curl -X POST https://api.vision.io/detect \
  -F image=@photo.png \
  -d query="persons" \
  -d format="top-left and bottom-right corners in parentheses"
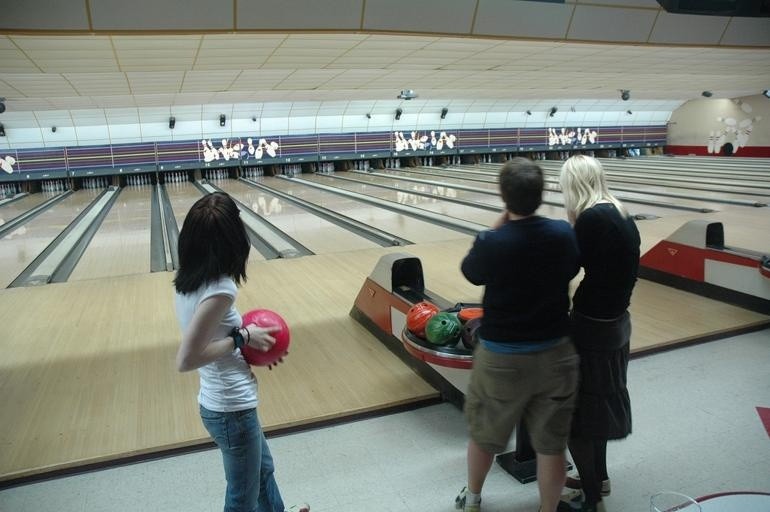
top-left (454, 156), bottom-right (581, 512)
top-left (552, 155), bottom-right (642, 512)
top-left (175, 191), bottom-right (310, 512)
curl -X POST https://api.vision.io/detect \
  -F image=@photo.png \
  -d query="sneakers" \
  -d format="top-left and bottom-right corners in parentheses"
top-left (538, 488), bottom-right (587, 512)
top-left (560, 486), bottom-right (608, 512)
top-left (284, 503), bottom-right (310, 512)
top-left (455, 486), bottom-right (482, 511)
top-left (563, 474), bottom-right (612, 496)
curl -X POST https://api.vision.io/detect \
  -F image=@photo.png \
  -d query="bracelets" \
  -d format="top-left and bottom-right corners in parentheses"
top-left (244, 327), bottom-right (251, 345)
top-left (226, 327), bottom-right (245, 349)
